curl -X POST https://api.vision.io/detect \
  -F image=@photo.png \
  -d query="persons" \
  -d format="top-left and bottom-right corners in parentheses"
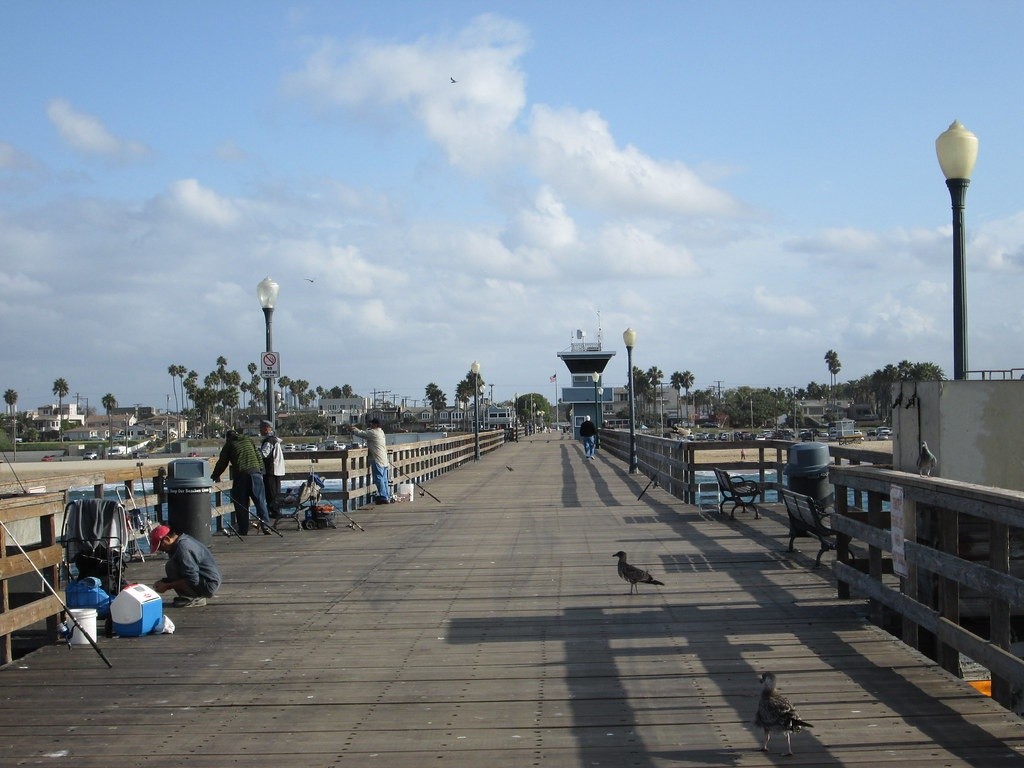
top-left (149, 525), bottom-right (221, 608)
top-left (258, 420), bottom-right (285, 518)
top-left (580, 415), bottom-right (596, 460)
top-left (350, 417), bottom-right (390, 503)
top-left (210, 430), bottom-right (272, 535)
top-left (740, 449), bottom-right (745, 460)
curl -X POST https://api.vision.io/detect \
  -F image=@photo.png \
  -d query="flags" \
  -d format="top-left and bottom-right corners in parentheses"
top-left (550, 374), bottom-right (556, 383)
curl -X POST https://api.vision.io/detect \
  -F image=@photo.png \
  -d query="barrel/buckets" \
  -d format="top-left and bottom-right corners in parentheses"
top-left (64, 608), bottom-right (98, 645)
top-left (400, 484), bottom-right (414, 501)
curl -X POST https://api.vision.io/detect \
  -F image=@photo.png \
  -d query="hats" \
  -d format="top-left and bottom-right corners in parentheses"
top-left (149, 525), bottom-right (170, 554)
top-left (257, 419), bottom-right (273, 428)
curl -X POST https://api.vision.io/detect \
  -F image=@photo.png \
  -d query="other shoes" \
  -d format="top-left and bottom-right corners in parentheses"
top-left (377, 499), bottom-right (390, 504)
top-left (234, 531), bottom-right (246, 536)
top-left (261, 527), bottom-right (272, 535)
top-left (184, 597), bottom-right (207, 607)
top-left (173, 596), bottom-right (190, 605)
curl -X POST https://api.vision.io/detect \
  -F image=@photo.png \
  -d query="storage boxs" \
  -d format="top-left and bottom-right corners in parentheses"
top-left (110, 583), bottom-right (163, 638)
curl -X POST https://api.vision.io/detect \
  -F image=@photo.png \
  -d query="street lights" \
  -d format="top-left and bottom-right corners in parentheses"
top-left (511, 397), bottom-right (515, 411)
top-left (934, 119), bottom-right (979, 381)
top-left (622, 327), bottom-right (638, 474)
top-left (598, 387), bottom-right (604, 428)
top-left (533, 403), bottom-right (536, 434)
top-left (591, 371), bottom-right (599, 448)
top-left (256, 275), bottom-right (280, 436)
top-left (479, 385), bottom-right (486, 431)
top-left (515, 393), bottom-right (520, 443)
top-left (471, 361), bottom-right (480, 460)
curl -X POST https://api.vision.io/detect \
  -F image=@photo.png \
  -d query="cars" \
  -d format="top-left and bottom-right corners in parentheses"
top-left (688, 426), bottom-right (836, 441)
top-left (854, 426), bottom-right (893, 441)
top-left (107, 448), bottom-right (123, 456)
top-left (88, 436), bottom-right (105, 441)
top-left (41, 455), bottom-right (52, 461)
top-left (82, 452), bottom-right (96, 460)
top-left (282, 440), bottom-right (368, 452)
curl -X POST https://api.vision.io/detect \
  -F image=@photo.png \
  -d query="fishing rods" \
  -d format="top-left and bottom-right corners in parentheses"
top-left (215, 485), bottom-right (284, 538)
top-left (1, 519), bottom-right (113, 670)
top-left (636, 391), bottom-right (717, 502)
top-left (284, 460), bottom-right (365, 532)
top-left (0, 445), bottom-right (77, 582)
top-left (356, 436), bottom-right (442, 503)
top-left (210, 504), bottom-right (244, 542)
top-left (136, 443), bottom-right (152, 532)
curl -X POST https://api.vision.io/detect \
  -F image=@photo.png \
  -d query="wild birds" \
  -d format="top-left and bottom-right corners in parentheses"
top-left (304, 277), bottom-right (317, 283)
top-left (530, 440), bottom-right (532, 443)
top-left (752, 672), bottom-right (815, 757)
top-left (505, 465), bottom-right (514, 472)
top-left (612, 551), bottom-right (665, 595)
top-left (450, 76), bottom-right (459, 83)
top-left (546, 440), bottom-right (550, 443)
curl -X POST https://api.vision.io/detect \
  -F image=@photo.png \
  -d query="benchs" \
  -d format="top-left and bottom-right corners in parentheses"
top-left (781, 488), bottom-right (855, 567)
top-left (836, 435), bottom-right (864, 445)
top-left (713, 467), bottom-right (761, 520)
